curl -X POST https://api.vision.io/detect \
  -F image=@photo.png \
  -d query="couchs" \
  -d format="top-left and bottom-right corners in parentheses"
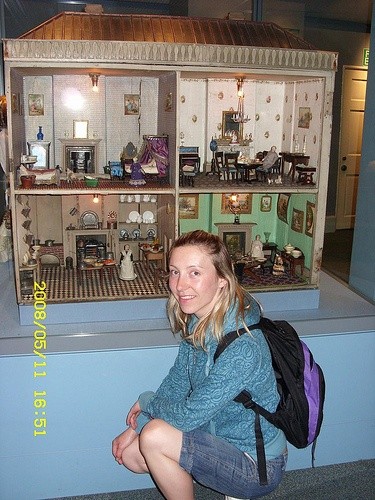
top-left (16, 164), bottom-right (61, 187)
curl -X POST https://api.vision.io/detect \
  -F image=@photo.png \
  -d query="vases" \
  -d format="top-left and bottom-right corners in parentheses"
top-left (37, 126), bottom-right (44, 140)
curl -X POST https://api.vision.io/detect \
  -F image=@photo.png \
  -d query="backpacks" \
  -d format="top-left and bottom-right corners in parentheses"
top-left (214, 316), bottom-right (327, 448)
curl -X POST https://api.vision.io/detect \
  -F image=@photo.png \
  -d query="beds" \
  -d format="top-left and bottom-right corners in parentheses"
top-left (123, 134), bottom-right (169, 183)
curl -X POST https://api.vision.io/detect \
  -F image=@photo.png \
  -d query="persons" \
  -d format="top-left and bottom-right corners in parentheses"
top-left (118, 244), bottom-right (137, 280)
top-left (112, 230), bottom-right (288, 500)
top-left (251, 234), bottom-right (265, 262)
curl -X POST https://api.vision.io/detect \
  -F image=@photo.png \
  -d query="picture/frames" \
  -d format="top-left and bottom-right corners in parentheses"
top-left (222, 110), bottom-right (243, 144)
top-left (276, 193), bottom-right (292, 225)
top-left (72, 119), bottom-right (89, 139)
top-left (28, 93), bottom-right (44, 116)
top-left (260, 195), bottom-right (272, 212)
top-left (297, 106), bottom-right (310, 129)
top-left (178, 193), bottom-right (199, 220)
top-left (290, 206), bottom-right (305, 233)
top-left (305, 200), bottom-right (316, 237)
top-left (221, 193), bottom-right (253, 214)
top-left (124, 94), bottom-right (139, 116)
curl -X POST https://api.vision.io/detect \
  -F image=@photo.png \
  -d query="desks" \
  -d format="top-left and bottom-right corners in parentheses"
top-left (27, 140), bottom-right (51, 170)
top-left (262, 242), bottom-right (278, 260)
top-left (19, 256), bottom-right (42, 297)
top-left (272, 246), bottom-right (305, 278)
top-left (231, 158), bottom-right (265, 184)
top-left (229, 255), bottom-right (267, 275)
top-left (212, 145), bottom-right (253, 175)
top-left (179, 145), bottom-right (200, 175)
top-left (279, 152), bottom-right (310, 183)
top-left (139, 247), bottom-right (164, 276)
top-left (78, 257), bottom-right (115, 286)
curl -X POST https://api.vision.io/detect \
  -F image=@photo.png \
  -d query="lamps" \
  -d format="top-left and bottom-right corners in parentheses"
top-left (231, 76), bottom-right (252, 123)
top-left (89, 72), bottom-right (100, 92)
top-left (21, 207), bottom-right (31, 219)
top-left (69, 206), bottom-right (79, 216)
top-left (93, 194), bottom-right (99, 203)
top-left (227, 193), bottom-right (242, 215)
top-left (25, 234), bottom-right (35, 245)
top-left (22, 220), bottom-right (32, 232)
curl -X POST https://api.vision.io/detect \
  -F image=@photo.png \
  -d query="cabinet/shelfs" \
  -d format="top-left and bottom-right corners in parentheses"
top-left (118, 201), bottom-right (159, 242)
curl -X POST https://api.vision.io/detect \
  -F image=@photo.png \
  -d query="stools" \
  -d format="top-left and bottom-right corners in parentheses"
top-left (295, 164), bottom-right (317, 186)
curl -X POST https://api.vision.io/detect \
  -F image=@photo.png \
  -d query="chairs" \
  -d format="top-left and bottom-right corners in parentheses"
top-left (153, 268), bottom-right (170, 292)
top-left (233, 262), bottom-right (246, 282)
top-left (256, 157), bottom-right (282, 182)
top-left (215, 151), bottom-right (234, 183)
top-left (108, 160), bottom-right (123, 181)
top-left (179, 155), bottom-right (202, 187)
top-left (223, 152), bottom-right (247, 186)
